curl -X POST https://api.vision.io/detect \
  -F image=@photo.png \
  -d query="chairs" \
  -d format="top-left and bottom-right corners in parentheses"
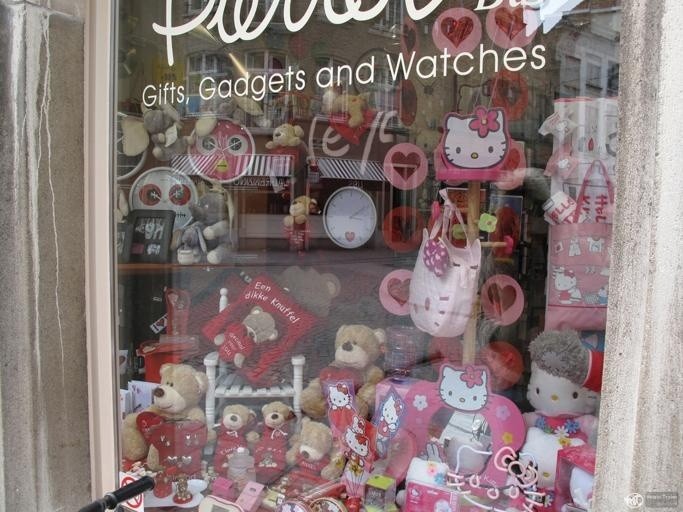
top-left (203, 286), bottom-right (306, 455)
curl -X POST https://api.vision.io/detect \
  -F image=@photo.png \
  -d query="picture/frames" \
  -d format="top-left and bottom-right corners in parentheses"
top-left (121, 209), bottom-right (175, 264)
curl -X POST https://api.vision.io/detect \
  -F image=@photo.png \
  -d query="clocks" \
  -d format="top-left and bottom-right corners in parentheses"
top-left (128, 166), bottom-right (199, 232)
top-left (322, 186), bottom-right (378, 251)
top-left (117, 112), bottom-right (147, 180)
top-left (187, 117), bottom-right (256, 184)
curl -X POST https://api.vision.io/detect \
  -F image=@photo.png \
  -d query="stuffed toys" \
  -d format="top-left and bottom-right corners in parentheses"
top-left (518, 329), bottom-right (603, 511)
top-left (121, 362), bottom-right (206, 481)
top-left (196, 88), bottom-right (264, 138)
top-left (139, 94), bottom-right (194, 163)
top-left (321, 88), bottom-right (370, 128)
top-left (117, 304), bottom-right (605, 511)
top-left (180, 190), bottom-right (233, 266)
top-left (208, 400), bottom-right (343, 512)
top-left (265, 124), bottom-right (309, 178)
top-left (299, 324), bottom-right (386, 420)
top-left (214, 305), bottom-right (279, 368)
top-left (283, 194), bottom-right (322, 252)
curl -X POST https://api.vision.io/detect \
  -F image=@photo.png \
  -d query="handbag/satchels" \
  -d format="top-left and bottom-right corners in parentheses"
top-left (543, 223), bottom-right (615, 333)
top-left (408, 231), bottom-right (482, 338)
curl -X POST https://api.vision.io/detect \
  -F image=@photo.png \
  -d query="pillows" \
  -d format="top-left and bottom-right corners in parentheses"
top-left (202, 275), bottom-right (317, 389)
